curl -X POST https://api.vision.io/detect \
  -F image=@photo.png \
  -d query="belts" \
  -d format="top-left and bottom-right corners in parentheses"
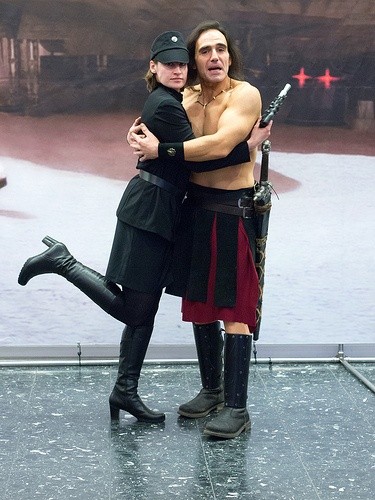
top-left (188, 196), bottom-right (256, 219)
top-left (138, 169), bottom-right (188, 204)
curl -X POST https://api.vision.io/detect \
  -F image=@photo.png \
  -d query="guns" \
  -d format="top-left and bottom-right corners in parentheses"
top-left (260, 83), bottom-right (291, 130)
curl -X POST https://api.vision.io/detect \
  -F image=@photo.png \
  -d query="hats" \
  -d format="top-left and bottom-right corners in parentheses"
top-left (150, 31), bottom-right (191, 63)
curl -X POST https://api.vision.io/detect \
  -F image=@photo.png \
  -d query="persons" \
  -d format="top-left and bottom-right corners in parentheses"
top-left (127, 20), bottom-right (262, 438)
top-left (18, 32), bottom-right (273, 425)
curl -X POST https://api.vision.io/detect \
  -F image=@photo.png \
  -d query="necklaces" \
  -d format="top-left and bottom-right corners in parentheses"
top-left (194, 79), bottom-right (235, 110)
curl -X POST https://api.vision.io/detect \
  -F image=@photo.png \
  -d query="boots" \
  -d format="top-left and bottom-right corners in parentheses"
top-left (177, 323), bottom-right (225, 418)
top-left (109, 324), bottom-right (165, 422)
top-left (203, 334), bottom-right (251, 436)
top-left (17, 236), bottom-right (121, 322)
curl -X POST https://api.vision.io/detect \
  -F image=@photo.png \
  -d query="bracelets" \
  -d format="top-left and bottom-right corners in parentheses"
top-left (158, 141), bottom-right (185, 162)
